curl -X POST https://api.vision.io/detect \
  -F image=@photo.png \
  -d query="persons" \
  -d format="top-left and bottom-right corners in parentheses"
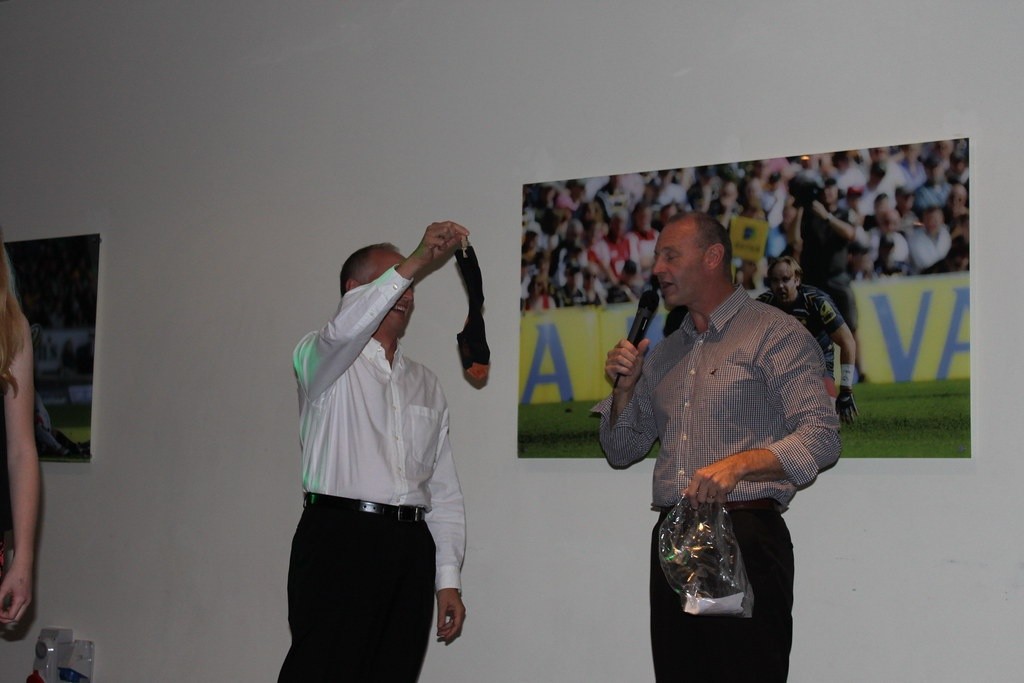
top-left (759, 251), bottom-right (859, 425)
top-left (589, 210), bottom-right (843, 681)
top-left (783, 171), bottom-right (871, 383)
top-left (521, 137), bottom-right (970, 310)
top-left (260, 219), bottom-right (473, 682)
top-left (1, 235), bottom-right (39, 633)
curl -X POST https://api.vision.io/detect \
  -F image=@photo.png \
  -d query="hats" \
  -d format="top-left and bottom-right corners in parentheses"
top-left (822, 172), bottom-right (837, 184)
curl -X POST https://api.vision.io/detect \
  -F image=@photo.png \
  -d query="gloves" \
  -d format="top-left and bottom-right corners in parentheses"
top-left (835, 385), bottom-right (858, 426)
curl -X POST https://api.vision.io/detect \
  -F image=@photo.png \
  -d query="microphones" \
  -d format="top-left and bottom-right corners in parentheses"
top-left (618, 290), bottom-right (659, 377)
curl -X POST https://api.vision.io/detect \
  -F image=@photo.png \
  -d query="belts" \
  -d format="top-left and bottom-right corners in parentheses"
top-left (304, 492), bottom-right (425, 521)
top-left (671, 497), bottom-right (772, 512)
